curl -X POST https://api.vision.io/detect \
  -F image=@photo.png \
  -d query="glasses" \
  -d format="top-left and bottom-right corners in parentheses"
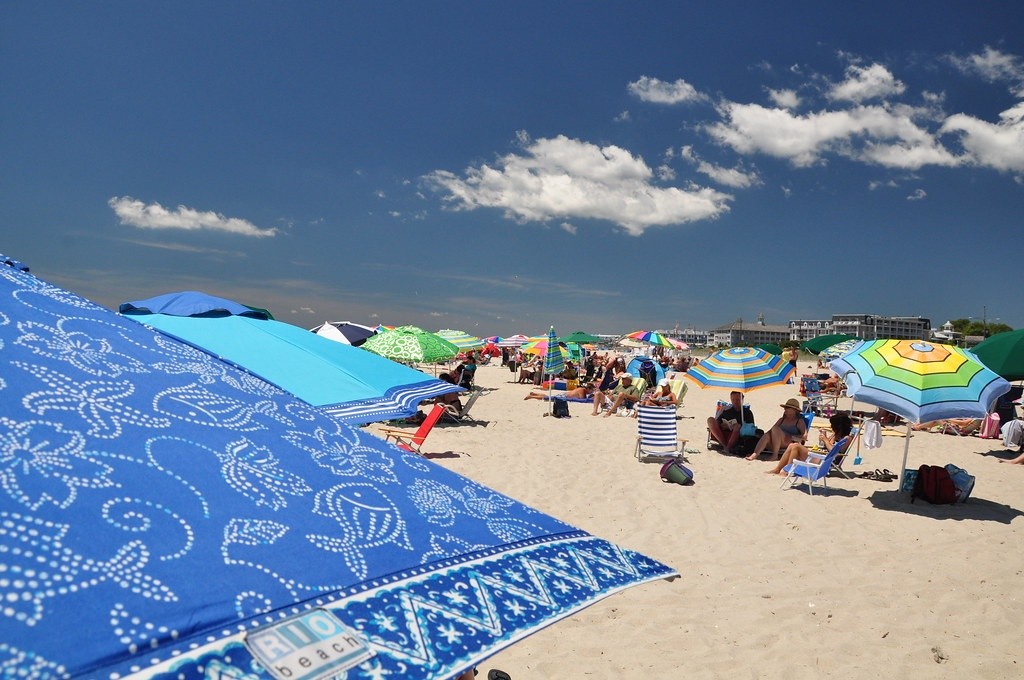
top-left (783, 406), bottom-right (790, 409)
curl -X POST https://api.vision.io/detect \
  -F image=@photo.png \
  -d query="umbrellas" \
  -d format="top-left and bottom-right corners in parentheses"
top-left (801, 333), bottom-right (1012, 495)
top-left (969, 329), bottom-right (1024, 383)
top-left (683, 346), bottom-right (797, 424)
top-left (1, 253), bottom-right (682, 679)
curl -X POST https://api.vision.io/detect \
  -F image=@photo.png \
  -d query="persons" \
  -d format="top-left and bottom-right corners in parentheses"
top-left (782, 347), bottom-right (791, 364)
top-left (439, 364), bottom-right (465, 385)
top-left (764, 413), bottom-right (853, 476)
top-left (872, 408), bottom-right (892, 422)
top-left (504, 348), bottom-right (754, 455)
top-left (788, 347), bottom-right (798, 377)
top-left (461, 356), bottom-right (477, 385)
top-left (906, 419), bottom-right (971, 436)
top-left (745, 398), bottom-right (807, 461)
top-left (819, 373), bottom-right (839, 392)
top-left (434, 373), bottom-right (462, 418)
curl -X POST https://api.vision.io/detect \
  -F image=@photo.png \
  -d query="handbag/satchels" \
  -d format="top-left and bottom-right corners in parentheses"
top-left (944, 462), bottom-right (975, 505)
top-left (902, 463), bottom-right (919, 493)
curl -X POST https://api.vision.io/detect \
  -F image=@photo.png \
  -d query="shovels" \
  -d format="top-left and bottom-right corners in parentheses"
top-left (854, 414), bottom-right (862, 465)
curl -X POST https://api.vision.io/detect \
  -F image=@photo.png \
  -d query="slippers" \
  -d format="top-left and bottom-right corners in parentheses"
top-left (684, 448), bottom-right (700, 453)
top-left (859, 468), bottom-right (898, 482)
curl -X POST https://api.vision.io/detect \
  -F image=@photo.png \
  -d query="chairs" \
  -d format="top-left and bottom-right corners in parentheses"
top-left (651, 379), bottom-right (689, 418)
top-left (457, 368), bottom-right (476, 391)
top-left (632, 402), bottom-right (691, 464)
top-left (705, 399), bottom-right (752, 449)
top-left (801, 377), bottom-right (841, 417)
top-left (440, 386), bottom-right (491, 428)
top-left (826, 422), bottom-right (867, 480)
top-left (778, 432), bottom-right (849, 494)
top-left (762, 410), bottom-right (819, 454)
top-left (377, 401), bottom-right (460, 460)
top-left (601, 377), bottom-right (649, 413)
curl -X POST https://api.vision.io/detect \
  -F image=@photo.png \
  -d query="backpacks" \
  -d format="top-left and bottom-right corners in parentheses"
top-left (553, 396), bottom-right (571, 418)
top-left (910, 464), bottom-right (957, 506)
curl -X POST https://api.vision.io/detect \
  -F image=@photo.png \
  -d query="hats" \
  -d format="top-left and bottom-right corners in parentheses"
top-left (780, 398), bottom-right (803, 412)
top-left (658, 378), bottom-right (670, 387)
top-left (621, 372), bottom-right (633, 381)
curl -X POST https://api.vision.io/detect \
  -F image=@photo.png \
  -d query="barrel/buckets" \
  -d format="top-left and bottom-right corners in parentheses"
top-left (659, 459), bottom-right (689, 483)
